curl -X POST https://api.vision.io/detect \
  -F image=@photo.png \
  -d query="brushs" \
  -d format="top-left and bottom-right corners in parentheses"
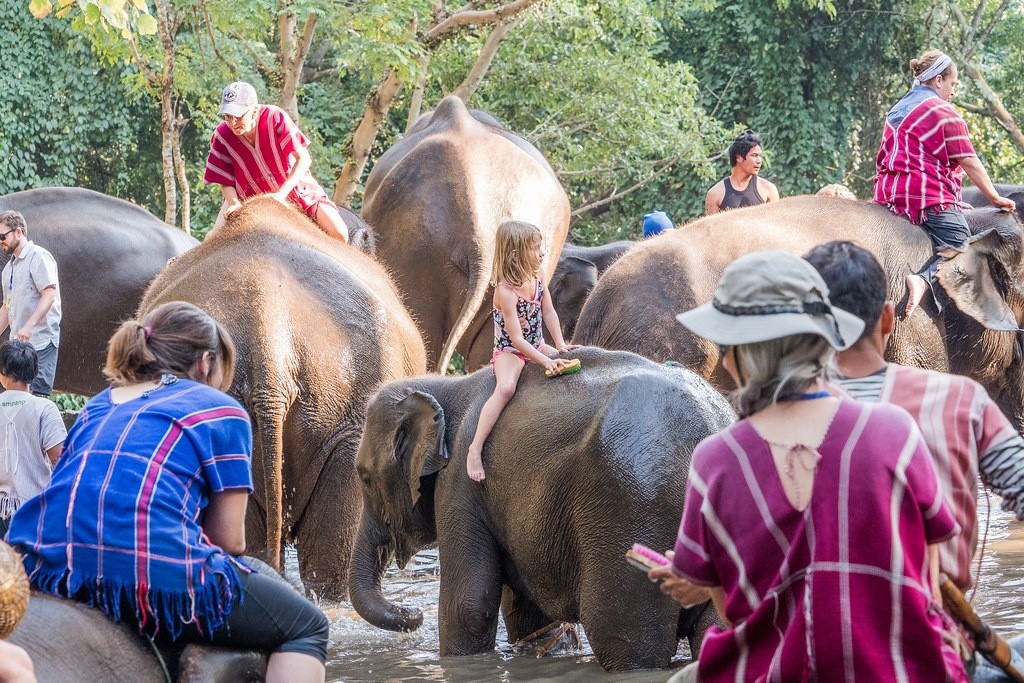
top-left (625, 543), bottom-right (671, 580)
top-left (1001, 206), bottom-right (1012, 212)
top-left (545, 358), bottom-right (581, 377)
top-left (225, 208), bottom-right (236, 220)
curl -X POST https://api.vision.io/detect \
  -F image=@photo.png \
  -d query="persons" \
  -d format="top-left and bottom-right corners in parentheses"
top-left (0, 638), bottom-right (37, 683)
top-left (466, 219), bottom-right (567, 482)
top-left (800, 242), bottom-right (1024, 673)
top-left (0, 339), bottom-right (68, 539)
top-left (0, 210), bottom-right (62, 398)
top-left (203, 81), bottom-right (349, 244)
top-left (705, 130), bottom-right (779, 217)
top-left (643, 210), bottom-right (674, 238)
top-left (648, 250), bottom-right (971, 683)
top-left (874, 50), bottom-right (1017, 318)
top-left (5, 302), bottom-right (329, 683)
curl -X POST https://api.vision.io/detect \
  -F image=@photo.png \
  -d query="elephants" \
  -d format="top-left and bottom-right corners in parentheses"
top-left (348, 347), bottom-right (743, 666)
top-left (0, 95), bottom-right (1024, 683)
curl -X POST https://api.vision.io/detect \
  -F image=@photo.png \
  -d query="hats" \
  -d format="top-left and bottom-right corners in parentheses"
top-left (217, 81), bottom-right (258, 117)
top-left (643, 210), bottom-right (674, 238)
top-left (676, 250), bottom-right (865, 351)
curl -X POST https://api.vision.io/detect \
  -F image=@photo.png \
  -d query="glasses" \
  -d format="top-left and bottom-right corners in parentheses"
top-left (719, 346), bottom-right (727, 356)
top-left (0, 229), bottom-right (16, 241)
top-left (221, 110), bottom-right (249, 121)
